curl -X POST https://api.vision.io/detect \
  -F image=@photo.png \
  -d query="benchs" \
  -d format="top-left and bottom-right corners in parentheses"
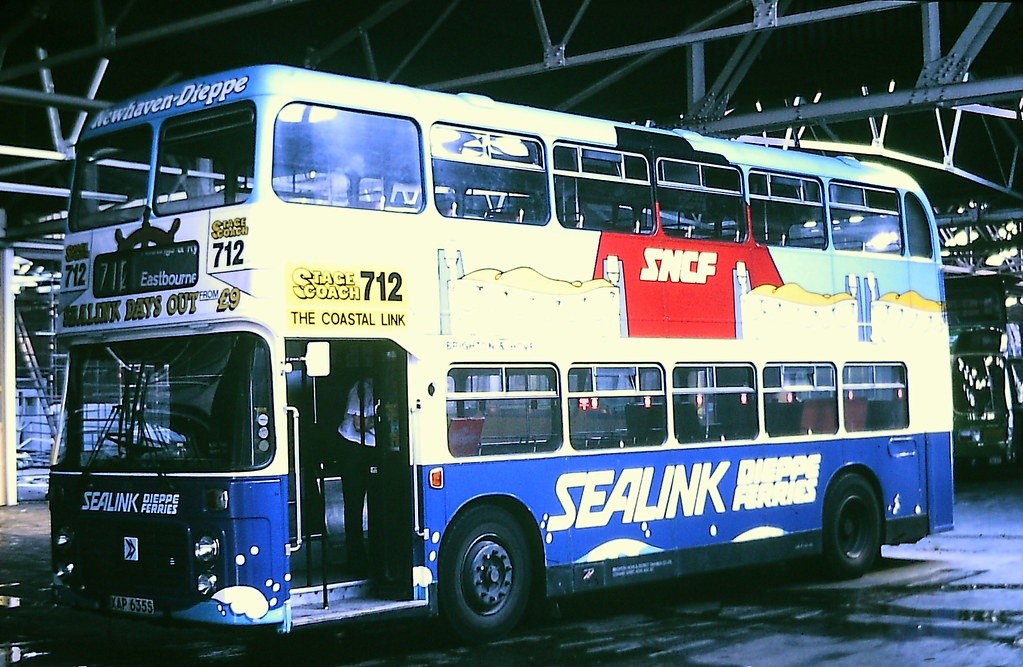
top-left (343, 191), bottom-right (901, 255)
top-left (446, 396), bottom-right (909, 457)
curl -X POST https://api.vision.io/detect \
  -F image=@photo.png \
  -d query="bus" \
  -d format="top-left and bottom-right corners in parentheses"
top-left (45, 64), bottom-right (957, 646)
top-left (940, 267), bottom-right (1023, 465)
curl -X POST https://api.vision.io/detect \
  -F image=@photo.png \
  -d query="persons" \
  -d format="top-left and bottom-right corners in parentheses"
top-left (338, 377), bottom-right (377, 559)
